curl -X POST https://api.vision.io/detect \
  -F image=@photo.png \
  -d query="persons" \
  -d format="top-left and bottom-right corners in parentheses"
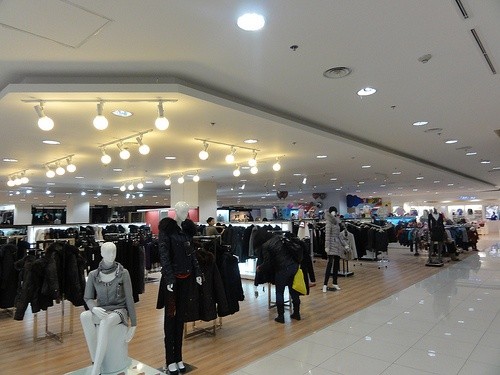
top-left (156, 201), bottom-right (202, 372)
top-left (80, 242), bottom-right (136, 375)
top-left (325, 206), bottom-right (351, 292)
top-left (206, 217), bottom-right (226, 235)
top-left (491, 212), bottom-right (499, 221)
top-left (428, 208), bottom-right (445, 263)
top-left (254, 230), bottom-right (303, 323)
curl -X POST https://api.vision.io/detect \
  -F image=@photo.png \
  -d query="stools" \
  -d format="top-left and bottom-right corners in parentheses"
top-left (94, 322), bottom-right (132, 373)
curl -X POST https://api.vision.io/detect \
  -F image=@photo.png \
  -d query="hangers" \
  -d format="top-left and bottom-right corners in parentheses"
top-left (366, 223), bottom-right (384, 233)
top-left (192, 238), bottom-right (210, 255)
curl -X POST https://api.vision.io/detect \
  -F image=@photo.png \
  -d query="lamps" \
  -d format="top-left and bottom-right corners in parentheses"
top-left (120, 179), bottom-right (144, 192)
top-left (233, 166), bottom-right (241, 178)
top-left (7, 168), bottom-right (29, 186)
top-left (198, 143), bottom-right (209, 160)
top-left (34, 101), bottom-right (55, 131)
top-left (164, 169), bottom-right (200, 185)
top-left (250, 165), bottom-right (258, 175)
top-left (273, 161), bottom-right (280, 171)
top-left (154, 100), bottom-right (170, 131)
top-left (117, 140), bottom-right (131, 160)
top-left (43, 154), bottom-right (76, 178)
top-left (99, 147), bottom-right (112, 165)
top-left (135, 134), bottom-right (152, 155)
top-left (247, 150), bottom-right (259, 167)
top-left (226, 147), bottom-right (237, 164)
top-left (92, 102), bottom-right (109, 131)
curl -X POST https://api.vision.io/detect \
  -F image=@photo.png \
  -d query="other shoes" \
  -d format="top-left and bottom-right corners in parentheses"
top-left (275, 314), bottom-right (284, 323)
top-left (291, 311), bottom-right (300, 319)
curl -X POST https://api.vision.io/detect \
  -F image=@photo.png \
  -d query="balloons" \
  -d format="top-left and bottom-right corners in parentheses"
top-left (277, 191), bottom-right (289, 201)
top-left (310, 193), bottom-right (327, 208)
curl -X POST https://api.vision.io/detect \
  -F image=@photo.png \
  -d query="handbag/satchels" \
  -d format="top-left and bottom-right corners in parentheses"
top-left (279, 235), bottom-right (304, 265)
top-left (292, 264), bottom-right (307, 295)
